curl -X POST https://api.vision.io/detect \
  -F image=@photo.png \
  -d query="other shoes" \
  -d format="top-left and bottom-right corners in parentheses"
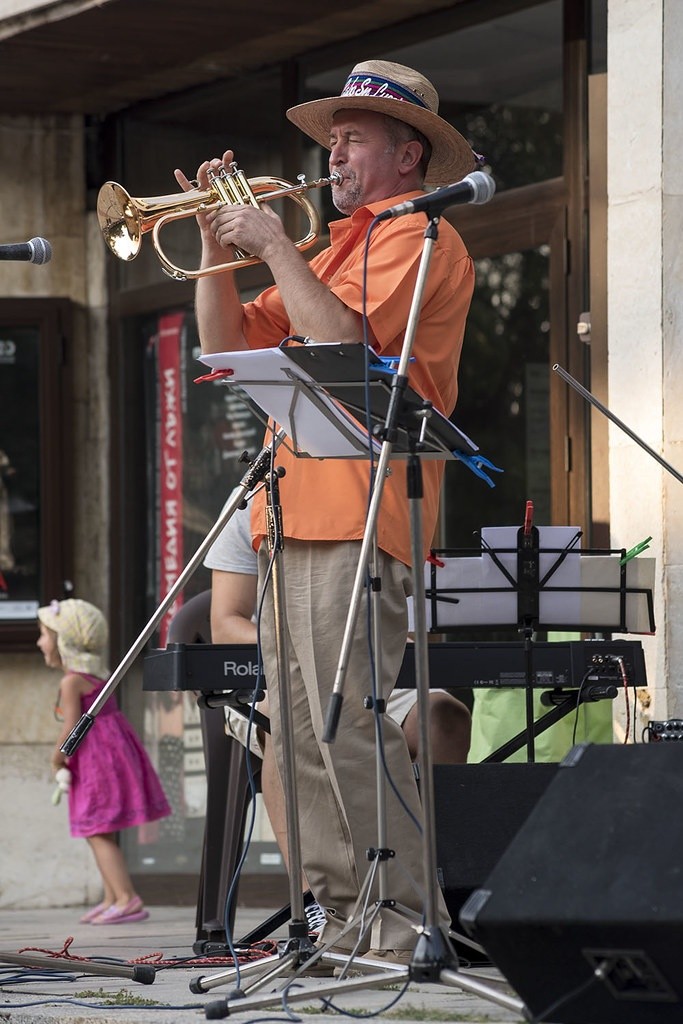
top-left (332, 949), bottom-right (414, 979)
top-left (277, 942), bottom-right (366, 978)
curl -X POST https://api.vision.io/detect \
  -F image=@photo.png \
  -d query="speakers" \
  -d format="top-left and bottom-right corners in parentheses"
top-left (411, 737), bottom-right (683, 1024)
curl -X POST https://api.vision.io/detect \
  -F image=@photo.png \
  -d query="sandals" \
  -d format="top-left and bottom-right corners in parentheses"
top-left (91, 896), bottom-right (149, 925)
top-left (79, 903), bottom-right (110, 924)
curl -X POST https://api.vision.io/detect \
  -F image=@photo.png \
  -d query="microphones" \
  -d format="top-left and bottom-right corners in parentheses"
top-left (0, 237), bottom-right (52, 265)
top-left (376, 170), bottom-right (496, 221)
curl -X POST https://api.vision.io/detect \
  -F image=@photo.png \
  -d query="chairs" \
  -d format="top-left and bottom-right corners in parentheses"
top-left (169, 589), bottom-right (273, 952)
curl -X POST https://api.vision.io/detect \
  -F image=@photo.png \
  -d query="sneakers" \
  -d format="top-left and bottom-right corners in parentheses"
top-left (297, 890), bottom-right (327, 939)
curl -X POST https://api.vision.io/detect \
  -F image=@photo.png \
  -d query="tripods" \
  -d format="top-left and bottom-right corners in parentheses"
top-left (188, 342), bottom-right (532, 1021)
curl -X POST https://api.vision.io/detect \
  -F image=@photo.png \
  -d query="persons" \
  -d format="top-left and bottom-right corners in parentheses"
top-left (201, 478), bottom-right (470, 938)
top-left (35, 599), bottom-right (173, 925)
top-left (172, 60), bottom-right (475, 982)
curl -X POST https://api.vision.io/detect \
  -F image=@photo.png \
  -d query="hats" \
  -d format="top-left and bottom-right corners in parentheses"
top-left (286, 60), bottom-right (475, 187)
top-left (37, 598), bottom-right (112, 681)
